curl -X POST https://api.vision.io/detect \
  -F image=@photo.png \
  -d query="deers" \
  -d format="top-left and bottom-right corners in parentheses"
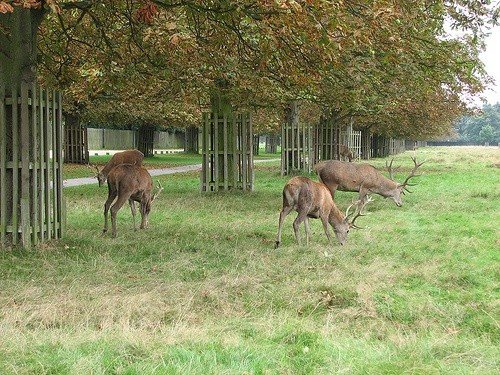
top-left (85, 149), bottom-right (144, 187)
top-left (276, 175), bottom-right (376, 247)
top-left (314, 153), bottom-right (425, 215)
top-left (102, 163), bottom-right (165, 239)
